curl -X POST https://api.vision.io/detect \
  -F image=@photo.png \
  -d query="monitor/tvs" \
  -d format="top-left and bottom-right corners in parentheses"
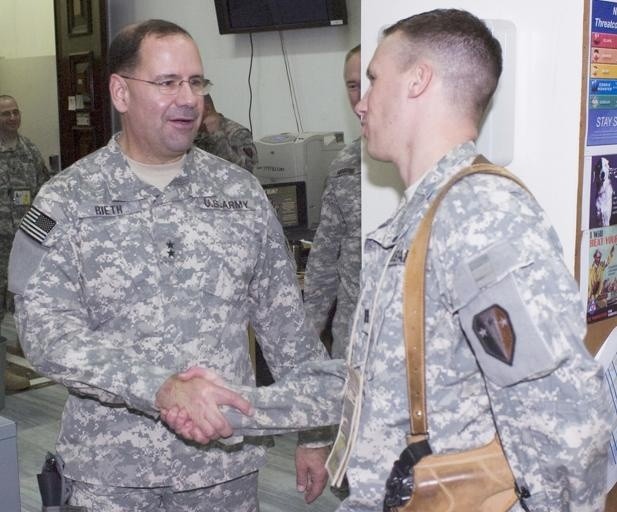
top-left (214, 0), bottom-right (348, 35)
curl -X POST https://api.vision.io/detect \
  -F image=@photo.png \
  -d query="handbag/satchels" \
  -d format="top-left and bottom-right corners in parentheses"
top-left (383, 440), bottom-right (522, 512)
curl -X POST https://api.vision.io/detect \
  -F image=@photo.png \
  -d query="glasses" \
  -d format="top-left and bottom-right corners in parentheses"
top-left (123, 76), bottom-right (214, 96)
top-left (0, 110), bottom-right (21, 117)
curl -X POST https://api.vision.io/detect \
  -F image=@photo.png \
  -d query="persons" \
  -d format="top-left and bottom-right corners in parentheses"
top-left (159, 10), bottom-right (612, 511)
top-left (1, 94), bottom-right (52, 391)
top-left (7, 20), bottom-right (336, 511)
